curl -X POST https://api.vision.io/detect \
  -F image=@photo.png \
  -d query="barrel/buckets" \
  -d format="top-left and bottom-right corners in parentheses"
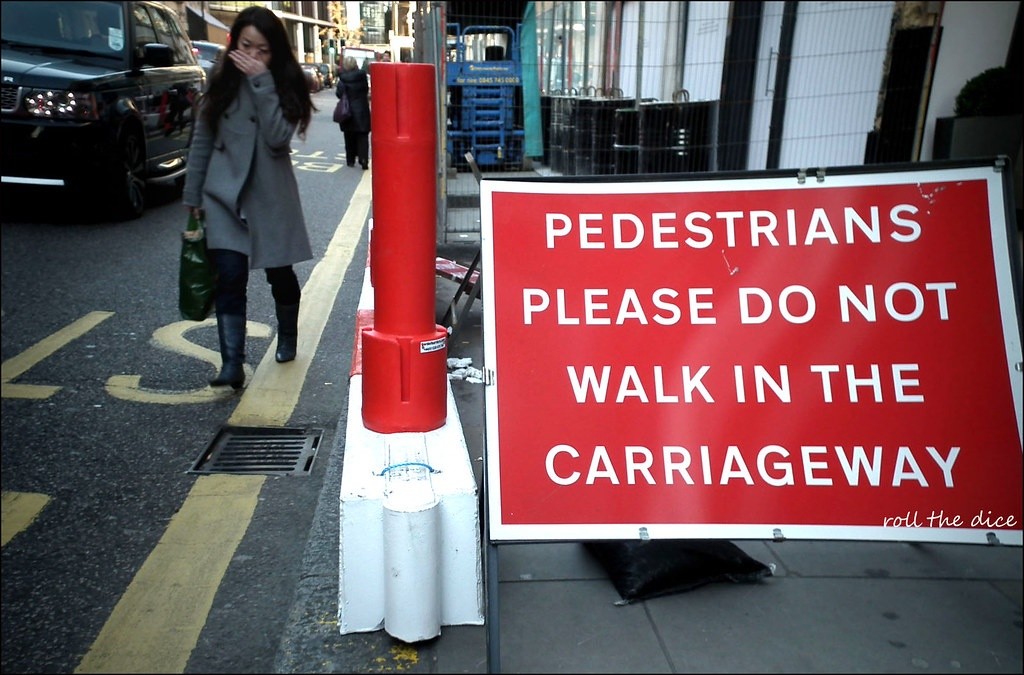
top-left (485, 46), bottom-right (504, 61)
top-left (541, 96), bottom-right (549, 166)
top-left (638, 89), bottom-right (716, 174)
top-left (560, 98), bottom-right (574, 175)
top-left (550, 97), bottom-right (560, 171)
top-left (591, 98), bottom-right (636, 174)
top-left (613, 108), bottom-right (640, 174)
top-left (573, 98), bottom-right (593, 174)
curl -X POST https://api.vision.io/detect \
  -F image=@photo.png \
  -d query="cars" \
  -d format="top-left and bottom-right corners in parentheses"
top-left (189, 41), bottom-right (226, 76)
top-left (299, 63), bottom-right (327, 92)
top-left (0, 0), bottom-right (207, 220)
top-left (314, 63), bottom-right (333, 88)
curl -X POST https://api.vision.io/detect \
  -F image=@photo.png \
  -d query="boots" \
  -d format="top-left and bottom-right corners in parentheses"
top-left (275, 301), bottom-right (297, 363)
top-left (209, 315), bottom-right (245, 389)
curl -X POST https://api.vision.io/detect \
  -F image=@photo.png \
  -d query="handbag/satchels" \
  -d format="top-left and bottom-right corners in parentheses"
top-left (334, 95), bottom-right (351, 123)
top-left (178, 210), bottom-right (216, 322)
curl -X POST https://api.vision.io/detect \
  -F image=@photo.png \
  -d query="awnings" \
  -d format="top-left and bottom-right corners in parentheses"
top-left (186, 1), bottom-right (230, 32)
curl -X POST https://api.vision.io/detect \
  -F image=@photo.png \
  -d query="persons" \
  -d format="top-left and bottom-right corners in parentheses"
top-left (383, 51), bottom-right (390, 62)
top-left (335, 55), bottom-right (370, 170)
top-left (182, 6), bottom-right (313, 391)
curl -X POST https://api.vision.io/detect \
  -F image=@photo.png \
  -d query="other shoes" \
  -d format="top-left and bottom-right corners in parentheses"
top-left (362, 159), bottom-right (368, 170)
